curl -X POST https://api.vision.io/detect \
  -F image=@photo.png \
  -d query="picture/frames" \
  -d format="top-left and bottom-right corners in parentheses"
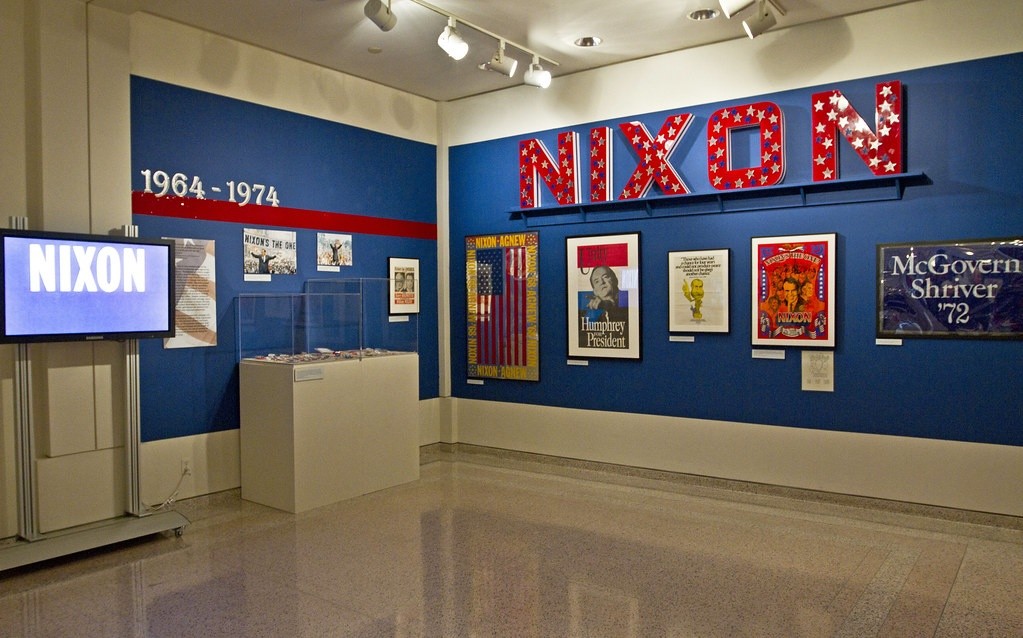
top-left (751, 232), bottom-right (838, 351)
top-left (565, 231), bottom-right (643, 360)
top-left (387, 256), bottom-right (420, 316)
top-left (667, 247), bottom-right (730, 335)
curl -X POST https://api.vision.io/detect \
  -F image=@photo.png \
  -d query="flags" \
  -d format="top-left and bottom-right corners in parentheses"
top-left (477, 247), bottom-right (527, 366)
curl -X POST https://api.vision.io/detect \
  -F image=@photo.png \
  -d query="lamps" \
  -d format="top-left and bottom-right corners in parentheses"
top-left (719, 0), bottom-right (755, 19)
top-left (364, 0), bottom-right (397, 31)
top-left (524, 55), bottom-right (551, 88)
top-left (438, 16), bottom-right (468, 60)
top-left (742, 0), bottom-right (778, 39)
top-left (490, 39), bottom-right (518, 77)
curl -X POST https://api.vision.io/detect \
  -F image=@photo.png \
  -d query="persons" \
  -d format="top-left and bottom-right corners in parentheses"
top-left (585, 266), bottom-right (628, 322)
top-left (395, 272), bottom-right (414, 292)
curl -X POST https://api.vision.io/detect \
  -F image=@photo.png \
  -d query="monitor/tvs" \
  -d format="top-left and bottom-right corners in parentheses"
top-left (0, 228), bottom-right (176, 344)
